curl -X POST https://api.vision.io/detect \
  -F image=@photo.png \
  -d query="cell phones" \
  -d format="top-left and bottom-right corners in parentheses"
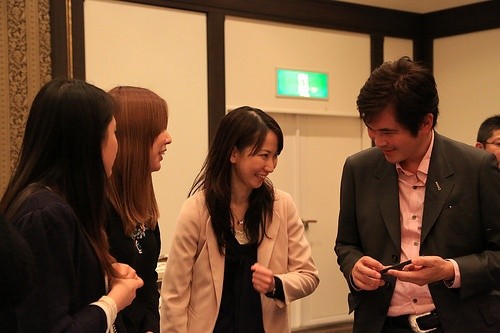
top-left (380, 260), bottom-right (412, 275)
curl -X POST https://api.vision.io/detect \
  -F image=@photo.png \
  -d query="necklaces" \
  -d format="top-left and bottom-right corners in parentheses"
top-left (229, 206), bottom-right (250, 225)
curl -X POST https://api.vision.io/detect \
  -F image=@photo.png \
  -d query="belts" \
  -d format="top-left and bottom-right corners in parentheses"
top-left (382, 308), bottom-right (441, 333)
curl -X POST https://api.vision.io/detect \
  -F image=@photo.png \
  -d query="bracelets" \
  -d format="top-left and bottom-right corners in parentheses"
top-left (265, 275), bottom-right (276, 295)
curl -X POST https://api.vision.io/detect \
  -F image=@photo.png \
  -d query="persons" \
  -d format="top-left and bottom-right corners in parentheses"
top-left (0, 78), bottom-right (144, 333)
top-left (334, 56), bottom-right (500, 333)
top-left (160, 105), bottom-right (320, 332)
top-left (475, 114), bottom-right (500, 166)
top-left (93, 85), bottom-right (172, 333)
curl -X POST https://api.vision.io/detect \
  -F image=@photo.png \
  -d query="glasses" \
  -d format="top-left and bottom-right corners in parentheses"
top-left (480, 141), bottom-right (500, 147)
